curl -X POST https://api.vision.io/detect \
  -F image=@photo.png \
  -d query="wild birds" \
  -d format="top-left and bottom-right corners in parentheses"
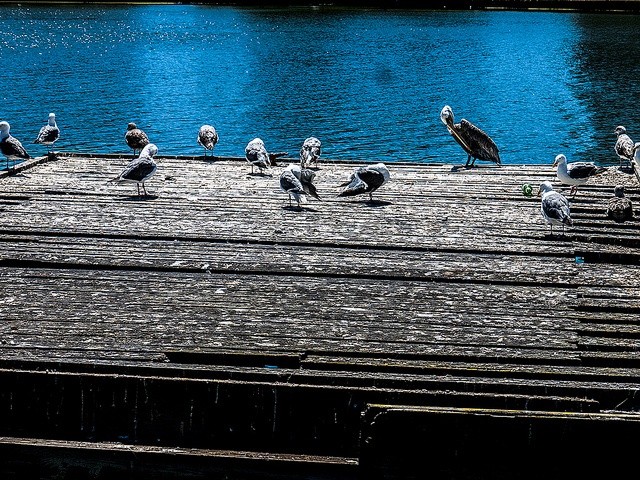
top-left (633, 142), bottom-right (640, 166)
top-left (279, 164), bottom-right (323, 210)
top-left (553, 154), bottom-right (608, 198)
top-left (196, 124), bottom-right (219, 159)
top-left (106, 143), bottom-right (157, 198)
top-left (245, 138), bottom-right (271, 174)
top-left (537, 181), bottom-right (574, 236)
top-left (441, 104), bottom-right (501, 169)
top-left (605, 185), bottom-right (633, 221)
top-left (613, 124), bottom-right (635, 169)
top-left (0, 120), bottom-right (35, 171)
top-left (301, 137), bottom-right (322, 168)
top-left (336, 162), bottom-right (389, 203)
top-left (125, 122), bottom-right (149, 156)
top-left (31, 112), bottom-right (60, 154)
